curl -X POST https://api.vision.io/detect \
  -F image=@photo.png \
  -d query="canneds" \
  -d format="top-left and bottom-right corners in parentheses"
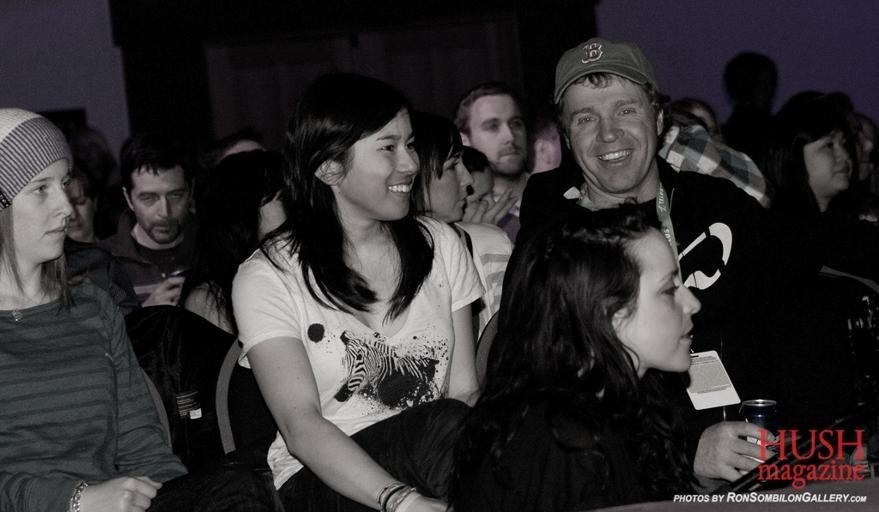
top-left (171, 267), bottom-right (190, 279)
top-left (736, 398), bottom-right (778, 472)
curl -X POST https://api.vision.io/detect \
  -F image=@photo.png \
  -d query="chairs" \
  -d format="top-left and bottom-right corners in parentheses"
top-left (215, 335), bottom-right (278, 466)
top-left (474, 313), bottom-right (506, 393)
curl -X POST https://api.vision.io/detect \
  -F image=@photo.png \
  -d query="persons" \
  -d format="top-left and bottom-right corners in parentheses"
top-left (1, 38), bottom-right (879, 512)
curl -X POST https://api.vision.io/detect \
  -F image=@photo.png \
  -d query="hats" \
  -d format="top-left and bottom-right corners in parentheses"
top-left (0, 106), bottom-right (74, 212)
top-left (553, 37), bottom-right (661, 106)
